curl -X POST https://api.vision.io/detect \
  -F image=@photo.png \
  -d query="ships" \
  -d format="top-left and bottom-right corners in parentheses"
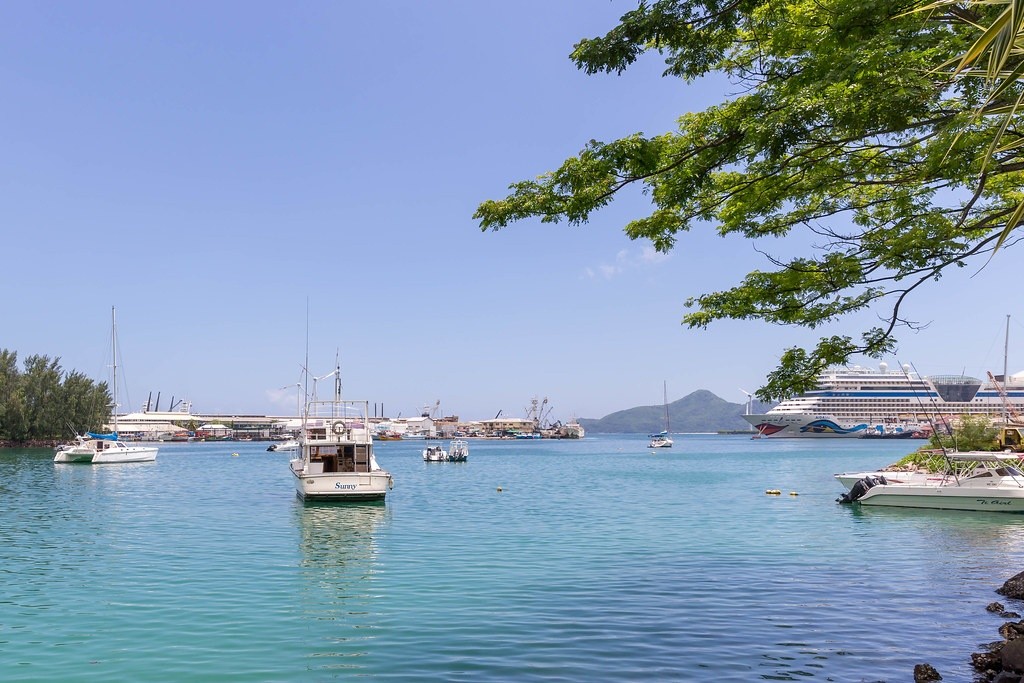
top-left (740, 358), bottom-right (1024, 439)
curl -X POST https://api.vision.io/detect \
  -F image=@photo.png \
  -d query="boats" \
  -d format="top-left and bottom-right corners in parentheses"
top-left (422, 442), bottom-right (448, 461)
top-left (447, 440), bottom-right (469, 462)
top-left (835, 361), bottom-right (1024, 513)
top-left (118, 434), bottom-right (136, 441)
top-left (266, 438), bottom-right (300, 452)
top-left (162, 427), bottom-right (585, 442)
top-left (289, 400), bottom-right (393, 503)
top-left (766, 489), bottom-right (781, 494)
top-left (790, 492), bottom-right (799, 495)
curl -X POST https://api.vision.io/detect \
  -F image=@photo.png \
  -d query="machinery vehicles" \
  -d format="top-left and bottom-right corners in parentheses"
top-left (986, 371), bottom-right (1024, 453)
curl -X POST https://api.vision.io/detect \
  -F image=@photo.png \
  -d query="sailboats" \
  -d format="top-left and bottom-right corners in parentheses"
top-left (53, 307), bottom-right (159, 463)
top-left (647, 380), bottom-right (672, 448)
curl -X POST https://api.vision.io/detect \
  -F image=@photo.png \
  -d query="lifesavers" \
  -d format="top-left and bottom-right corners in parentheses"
top-left (332, 420), bottom-right (346, 434)
